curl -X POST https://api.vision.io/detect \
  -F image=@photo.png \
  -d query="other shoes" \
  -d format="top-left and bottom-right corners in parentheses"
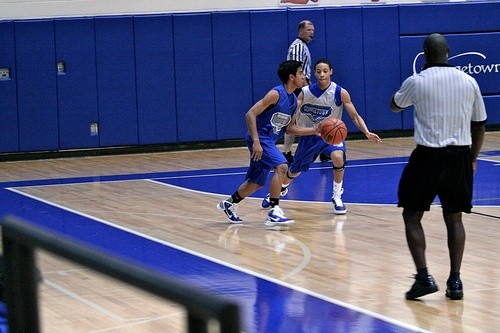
top-left (445, 279), bottom-right (464, 299)
top-left (282, 151), bottom-right (294, 164)
top-left (405, 273), bottom-right (439, 300)
top-left (320, 152), bottom-right (332, 161)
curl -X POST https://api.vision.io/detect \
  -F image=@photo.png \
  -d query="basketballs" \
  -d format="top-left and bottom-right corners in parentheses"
top-left (319, 118), bottom-right (347, 145)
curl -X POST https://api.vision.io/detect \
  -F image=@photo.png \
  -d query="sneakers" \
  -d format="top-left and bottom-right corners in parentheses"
top-left (265, 205), bottom-right (296, 226)
top-left (217, 200), bottom-right (244, 223)
top-left (261, 188), bottom-right (288, 208)
top-left (330, 188), bottom-right (347, 213)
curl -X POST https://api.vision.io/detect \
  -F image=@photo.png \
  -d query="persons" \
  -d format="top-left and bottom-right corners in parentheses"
top-left (389, 34), bottom-right (488, 300)
top-left (216, 60), bottom-right (323, 226)
top-left (281, 20), bottom-right (332, 164)
top-left (261, 58), bottom-right (382, 215)
